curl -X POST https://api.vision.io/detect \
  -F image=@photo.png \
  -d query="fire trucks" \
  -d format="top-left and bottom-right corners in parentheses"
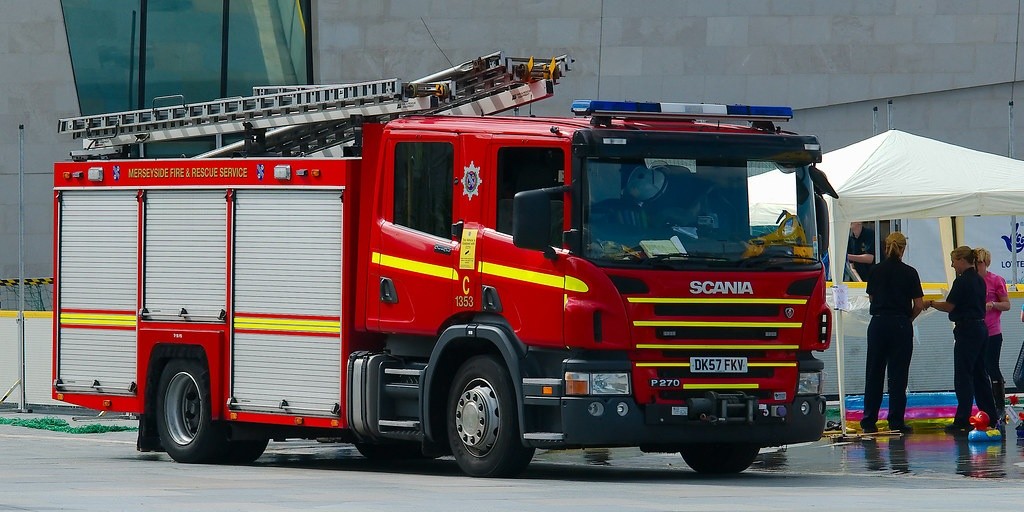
top-left (50, 51), bottom-right (839, 477)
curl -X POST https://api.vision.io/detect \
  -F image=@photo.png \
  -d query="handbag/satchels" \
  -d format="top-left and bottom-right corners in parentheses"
top-left (740, 210), bottom-right (806, 261)
top-left (1013, 341), bottom-right (1024, 392)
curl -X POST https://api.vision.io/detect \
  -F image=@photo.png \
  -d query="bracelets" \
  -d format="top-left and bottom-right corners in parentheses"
top-left (992, 300), bottom-right (996, 307)
top-left (929, 299), bottom-right (933, 307)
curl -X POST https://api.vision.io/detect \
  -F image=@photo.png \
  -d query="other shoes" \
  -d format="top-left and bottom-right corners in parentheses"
top-left (944, 424), bottom-right (973, 433)
top-left (891, 425), bottom-right (914, 434)
top-left (863, 424), bottom-right (879, 434)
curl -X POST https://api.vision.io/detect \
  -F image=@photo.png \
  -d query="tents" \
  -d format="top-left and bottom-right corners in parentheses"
top-left (747, 123), bottom-right (1024, 437)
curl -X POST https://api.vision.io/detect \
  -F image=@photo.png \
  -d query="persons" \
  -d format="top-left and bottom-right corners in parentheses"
top-left (1013, 289), bottom-right (1024, 437)
top-left (920, 244), bottom-right (1010, 435)
top-left (861, 231), bottom-right (924, 435)
top-left (847, 222), bottom-right (876, 282)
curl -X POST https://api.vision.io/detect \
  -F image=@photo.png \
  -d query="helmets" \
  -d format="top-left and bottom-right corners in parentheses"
top-left (626, 165), bottom-right (669, 207)
